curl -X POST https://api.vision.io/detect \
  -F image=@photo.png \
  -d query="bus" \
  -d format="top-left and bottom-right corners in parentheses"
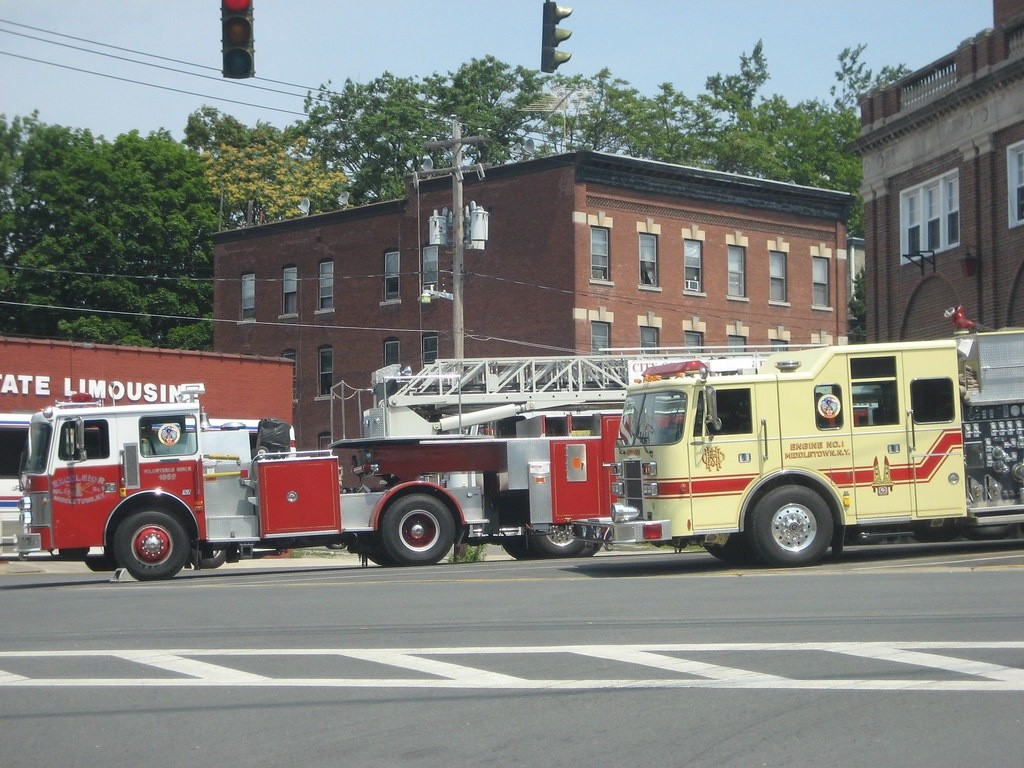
top-left (0, 412), bottom-right (297, 569)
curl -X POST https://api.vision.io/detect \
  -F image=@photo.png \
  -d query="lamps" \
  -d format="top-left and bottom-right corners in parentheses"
top-left (960, 244), bottom-right (978, 277)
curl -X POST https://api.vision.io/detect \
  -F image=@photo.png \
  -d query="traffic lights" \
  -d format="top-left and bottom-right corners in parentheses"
top-left (220, 0), bottom-right (255, 79)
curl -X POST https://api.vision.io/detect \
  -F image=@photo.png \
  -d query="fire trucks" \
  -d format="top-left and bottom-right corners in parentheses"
top-left (570, 306), bottom-right (1024, 567)
top-left (14, 343), bottom-right (829, 581)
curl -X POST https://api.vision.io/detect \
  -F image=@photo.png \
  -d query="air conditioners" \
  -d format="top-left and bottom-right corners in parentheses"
top-left (685, 280), bottom-right (699, 291)
